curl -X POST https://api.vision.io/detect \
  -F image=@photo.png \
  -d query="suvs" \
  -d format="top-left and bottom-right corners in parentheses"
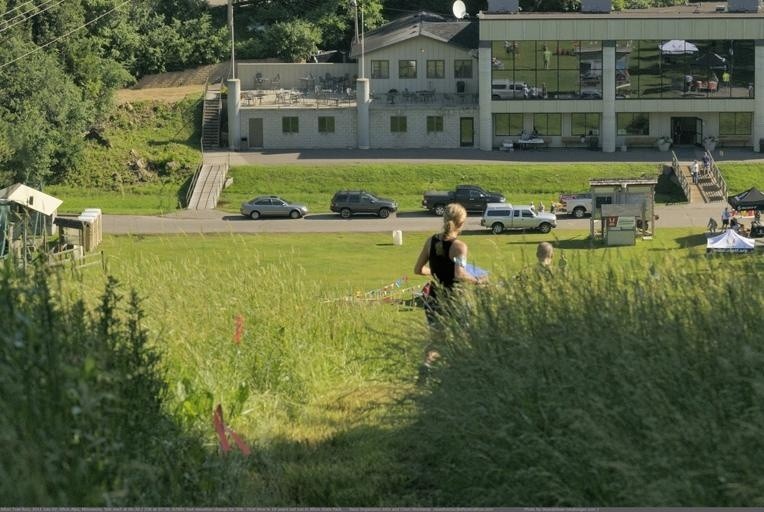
top-left (330, 190), bottom-right (398, 219)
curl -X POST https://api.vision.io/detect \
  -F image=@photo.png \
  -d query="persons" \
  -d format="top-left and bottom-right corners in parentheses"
top-left (685, 72), bottom-right (693, 92)
top-left (529, 201), bottom-right (535, 213)
top-left (538, 201), bottom-right (544, 214)
top-left (706, 218), bottom-right (717, 236)
top-left (502, 40), bottom-right (521, 59)
top-left (719, 207), bottom-right (730, 233)
top-left (413, 203), bottom-right (490, 387)
top-left (690, 159), bottom-right (700, 185)
top-left (550, 201), bottom-right (556, 214)
top-left (721, 70), bottom-right (730, 90)
top-left (516, 242), bottom-right (559, 288)
top-left (518, 85), bottom-right (530, 99)
top-left (702, 152), bottom-right (710, 177)
top-left (529, 85), bottom-right (538, 99)
top-left (539, 83), bottom-right (547, 99)
top-left (730, 216), bottom-right (740, 232)
top-left (541, 46), bottom-right (551, 70)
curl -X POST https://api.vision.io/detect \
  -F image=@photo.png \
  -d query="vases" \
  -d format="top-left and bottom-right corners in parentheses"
top-left (704, 139), bottom-right (716, 151)
top-left (657, 139), bottom-right (671, 152)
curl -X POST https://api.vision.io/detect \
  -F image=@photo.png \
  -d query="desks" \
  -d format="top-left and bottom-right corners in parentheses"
top-left (519, 139), bottom-right (544, 152)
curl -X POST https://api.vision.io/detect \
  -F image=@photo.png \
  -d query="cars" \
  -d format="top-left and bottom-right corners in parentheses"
top-left (240, 195), bottom-right (309, 220)
top-left (492, 81), bottom-right (603, 100)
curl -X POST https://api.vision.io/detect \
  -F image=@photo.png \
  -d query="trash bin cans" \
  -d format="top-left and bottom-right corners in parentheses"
top-left (589, 135), bottom-right (599, 153)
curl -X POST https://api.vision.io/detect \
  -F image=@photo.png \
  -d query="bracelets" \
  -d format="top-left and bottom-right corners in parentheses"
top-left (474, 275), bottom-right (480, 286)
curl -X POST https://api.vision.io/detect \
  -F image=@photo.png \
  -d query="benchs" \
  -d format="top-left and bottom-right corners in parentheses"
top-left (528, 137), bottom-right (553, 149)
top-left (562, 136), bottom-right (589, 150)
top-left (719, 135), bottom-right (751, 147)
top-left (624, 136), bottom-right (657, 149)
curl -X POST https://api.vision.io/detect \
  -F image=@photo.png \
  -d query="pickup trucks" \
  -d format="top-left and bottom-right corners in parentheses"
top-left (421, 184), bottom-right (506, 216)
top-left (566, 197), bottom-right (592, 218)
top-left (480, 202), bottom-right (558, 234)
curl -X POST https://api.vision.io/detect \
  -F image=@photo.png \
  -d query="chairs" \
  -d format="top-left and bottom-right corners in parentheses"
top-left (244, 73), bottom-right (478, 104)
